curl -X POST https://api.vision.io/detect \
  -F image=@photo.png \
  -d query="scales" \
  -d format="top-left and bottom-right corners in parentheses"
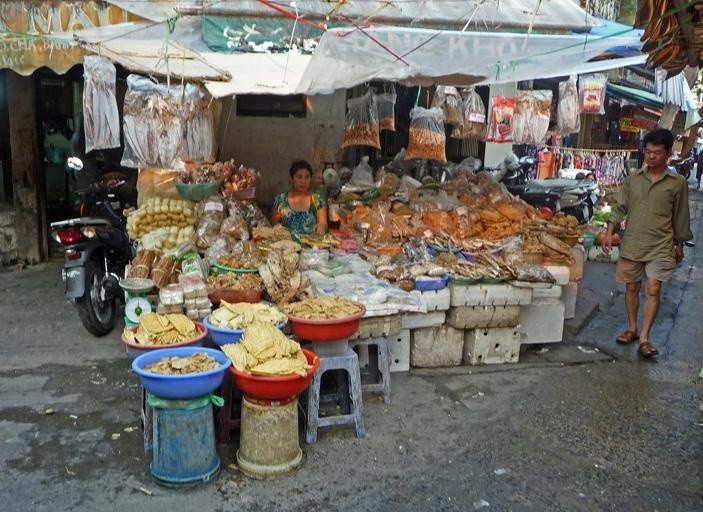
top-left (119, 278), bottom-right (154, 328)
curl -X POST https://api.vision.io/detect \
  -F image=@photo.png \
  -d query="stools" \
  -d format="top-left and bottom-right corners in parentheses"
top-left (140, 385), bottom-right (153, 454)
top-left (303, 343), bottom-right (366, 444)
top-left (348, 338), bottom-right (391, 413)
top-left (218, 374), bottom-right (242, 443)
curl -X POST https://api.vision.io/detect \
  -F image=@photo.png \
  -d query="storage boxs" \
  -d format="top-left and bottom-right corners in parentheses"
top-left (446, 305), bottom-right (519, 329)
top-left (358, 330), bottom-right (410, 373)
top-left (561, 282), bottom-right (577, 320)
top-left (348, 317), bottom-right (401, 340)
top-left (448, 281), bottom-right (533, 305)
top-left (409, 289), bottom-right (450, 312)
top-left (410, 328), bottom-right (465, 367)
top-left (563, 243), bottom-right (585, 281)
top-left (588, 244), bottom-right (620, 263)
top-left (465, 329), bottom-right (521, 365)
top-left (400, 311), bottom-right (446, 330)
top-left (515, 283), bottom-right (564, 344)
top-left (541, 266), bottom-right (570, 284)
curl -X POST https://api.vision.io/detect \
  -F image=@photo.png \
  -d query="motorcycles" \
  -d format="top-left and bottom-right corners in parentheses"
top-left (493, 150), bottom-right (597, 224)
top-left (670, 157), bottom-right (694, 180)
top-left (50, 154), bottom-right (134, 335)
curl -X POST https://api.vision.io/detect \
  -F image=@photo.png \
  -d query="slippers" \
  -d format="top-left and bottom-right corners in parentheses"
top-left (639, 343), bottom-right (659, 357)
top-left (616, 330), bottom-right (640, 343)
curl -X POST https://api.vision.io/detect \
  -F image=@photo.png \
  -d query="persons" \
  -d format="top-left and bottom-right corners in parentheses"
top-left (586, 174), bottom-right (596, 182)
top-left (602, 128), bottom-right (694, 358)
top-left (270, 159), bottom-right (329, 237)
top-left (694, 150), bottom-right (703, 189)
top-left (575, 172), bottom-right (586, 182)
